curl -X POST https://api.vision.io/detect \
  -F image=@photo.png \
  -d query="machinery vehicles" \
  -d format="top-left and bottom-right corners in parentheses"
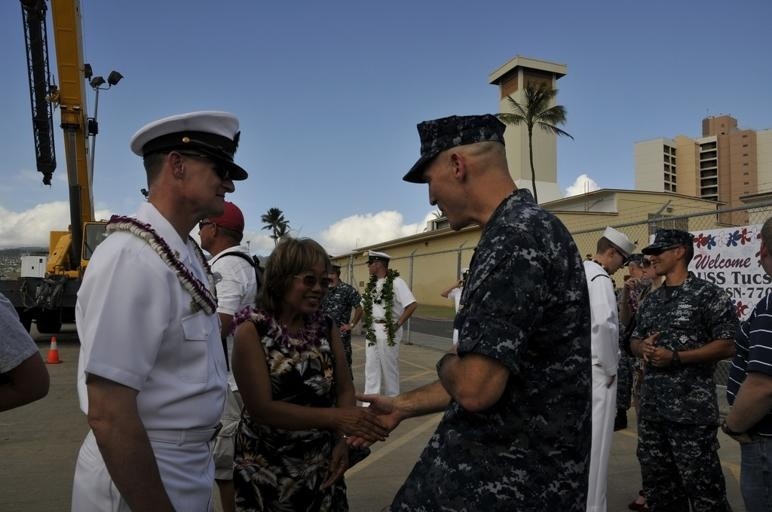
top-left (0, 1), bottom-right (109, 335)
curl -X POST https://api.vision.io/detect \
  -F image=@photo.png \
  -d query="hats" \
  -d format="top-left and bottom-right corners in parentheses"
top-left (368, 250), bottom-right (391, 263)
top-left (208, 200), bottom-right (244, 233)
top-left (602, 226), bottom-right (694, 267)
top-left (131, 111), bottom-right (248, 180)
top-left (331, 261), bottom-right (342, 272)
top-left (402, 114), bottom-right (506, 183)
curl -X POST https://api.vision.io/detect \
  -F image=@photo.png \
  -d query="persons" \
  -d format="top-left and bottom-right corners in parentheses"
top-left (440, 268), bottom-right (469, 344)
top-left (197, 202), bottom-right (418, 512)
top-left (70, 110), bottom-right (248, 512)
top-left (343, 115), bottom-right (590, 510)
top-left (1, 291), bottom-right (50, 412)
top-left (583, 217), bottom-right (772, 511)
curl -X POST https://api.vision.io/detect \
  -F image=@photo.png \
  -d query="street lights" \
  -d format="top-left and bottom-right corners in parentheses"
top-left (84, 64), bottom-right (124, 184)
top-left (246, 240), bottom-right (250, 250)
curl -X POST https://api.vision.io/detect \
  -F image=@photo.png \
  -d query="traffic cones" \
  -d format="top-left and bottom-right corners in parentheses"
top-left (44, 336), bottom-right (63, 364)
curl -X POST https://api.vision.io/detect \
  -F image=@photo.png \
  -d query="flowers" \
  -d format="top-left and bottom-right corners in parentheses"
top-left (229, 301), bottom-right (325, 355)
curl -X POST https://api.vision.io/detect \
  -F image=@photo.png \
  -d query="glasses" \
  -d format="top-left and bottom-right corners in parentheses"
top-left (293, 274), bottom-right (334, 289)
top-left (368, 259), bottom-right (386, 266)
top-left (199, 221), bottom-right (224, 231)
top-left (164, 146), bottom-right (230, 180)
